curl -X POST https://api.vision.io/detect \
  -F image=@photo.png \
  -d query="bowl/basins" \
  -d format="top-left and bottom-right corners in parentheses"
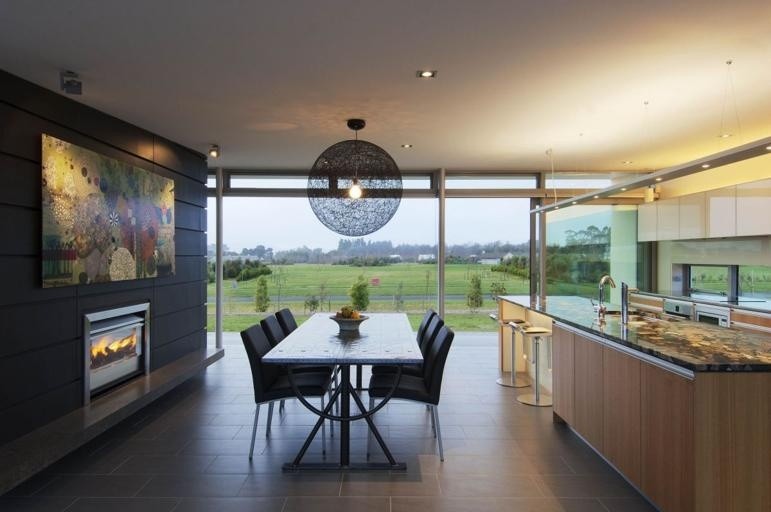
top-left (329, 314), bottom-right (370, 330)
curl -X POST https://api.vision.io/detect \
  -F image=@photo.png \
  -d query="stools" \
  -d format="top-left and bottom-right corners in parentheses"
top-left (488, 312), bottom-right (553, 408)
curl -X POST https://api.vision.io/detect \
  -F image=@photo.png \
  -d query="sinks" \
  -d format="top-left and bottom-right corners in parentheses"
top-left (605, 311), bottom-right (639, 315)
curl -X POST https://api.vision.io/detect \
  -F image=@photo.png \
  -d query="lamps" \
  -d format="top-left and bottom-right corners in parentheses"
top-left (306, 119), bottom-right (405, 240)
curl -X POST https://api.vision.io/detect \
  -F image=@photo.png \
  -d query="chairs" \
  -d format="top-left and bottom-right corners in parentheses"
top-left (241, 306), bottom-right (456, 473)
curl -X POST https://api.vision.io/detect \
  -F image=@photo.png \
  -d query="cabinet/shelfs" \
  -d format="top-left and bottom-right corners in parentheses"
top-left (708, 176), bottom-right (771, 239)
top-left (638, 202), bottom-right (657, 242)
top-left (656, 192), bottom-right (708, 242)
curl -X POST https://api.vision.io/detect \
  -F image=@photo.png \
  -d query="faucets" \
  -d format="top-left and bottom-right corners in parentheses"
top-left (599, 276), bottom-right (616, 305)
